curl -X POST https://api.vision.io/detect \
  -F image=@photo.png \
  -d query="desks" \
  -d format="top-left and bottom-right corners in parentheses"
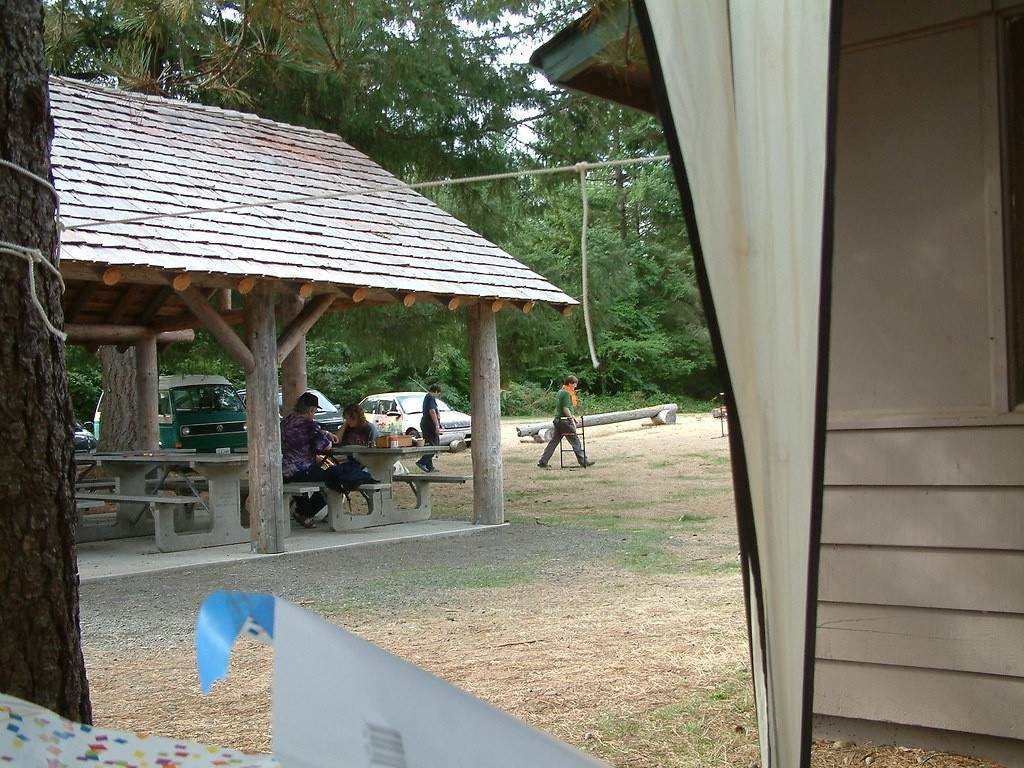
top-left (124, 448), bottom-right (197, 454)
top-left (318, 445), bottom-right (450, 524)
top-left (74, 454), bottom-right (122, 459)
top-left (96, 454), bottom-right (249, 548)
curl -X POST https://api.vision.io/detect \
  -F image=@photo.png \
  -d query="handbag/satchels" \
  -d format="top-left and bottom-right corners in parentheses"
top-left (316, 453), bottom-right (381, 495)
top-left (557, 416), bottom-right (576, 434)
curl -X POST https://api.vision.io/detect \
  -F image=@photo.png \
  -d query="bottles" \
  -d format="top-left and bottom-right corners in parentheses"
top-left (390, 428), bottom-right (398, 448)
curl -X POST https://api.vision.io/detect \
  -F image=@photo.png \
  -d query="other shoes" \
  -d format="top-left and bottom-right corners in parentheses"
top-left (292, 512), bottom-right (307, 526)
top-left (416, 461), bottom-right (429, 472)
top-left (537, 460), bottom-right (551, 467)
top-left (428, 467), bottom-right (440, 472)
top-left (580, 460), bottom-right (595, 466)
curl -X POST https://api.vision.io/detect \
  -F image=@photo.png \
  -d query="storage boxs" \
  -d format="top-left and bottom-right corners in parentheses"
top-left (375, 435), bottom-right (412, 448)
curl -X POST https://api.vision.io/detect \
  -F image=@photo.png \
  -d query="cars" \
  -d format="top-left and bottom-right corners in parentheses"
top-left (357, 391), bottom-right (472, 446)
top-left (73, 420), bottom-right (97, 452)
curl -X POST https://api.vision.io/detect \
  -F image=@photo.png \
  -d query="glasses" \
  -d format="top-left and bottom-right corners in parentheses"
top-left (343, 416), bottom-right (358, 422)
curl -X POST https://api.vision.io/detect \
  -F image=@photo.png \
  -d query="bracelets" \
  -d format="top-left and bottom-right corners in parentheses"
top-left (435, 426), bottom-right (439, 428)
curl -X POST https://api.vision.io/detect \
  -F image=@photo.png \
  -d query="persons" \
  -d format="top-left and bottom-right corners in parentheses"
top-left (333, 403), bottom-right (379, 481)
top-left (415, 385), bottom-right (441, 472)
top-left (280, 392), bottom-right (341, 529)
top-left (537, 375), bottom-right (595, 468)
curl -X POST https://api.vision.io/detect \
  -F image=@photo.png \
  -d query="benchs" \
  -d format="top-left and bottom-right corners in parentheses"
top-left (283, 484), bottom-right (319, 498)
top-left (74, 475), bottom-right (208, 488)
top-left (75, 490), bottom-right (202, 505)
top-left (304, 483), bottom-right (392, 490)
top-left (391, 472), bottom-right (473, 484)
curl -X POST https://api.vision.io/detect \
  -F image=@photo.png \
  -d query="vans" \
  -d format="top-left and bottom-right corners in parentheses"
top-left (92, 374), bottom-right (249, 451)
top-left (237, 385), bottom-right (347, 433)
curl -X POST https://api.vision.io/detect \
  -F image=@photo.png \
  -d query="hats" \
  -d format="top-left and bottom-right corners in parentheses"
top-left (300, 392), bottom-right (323, 409)
